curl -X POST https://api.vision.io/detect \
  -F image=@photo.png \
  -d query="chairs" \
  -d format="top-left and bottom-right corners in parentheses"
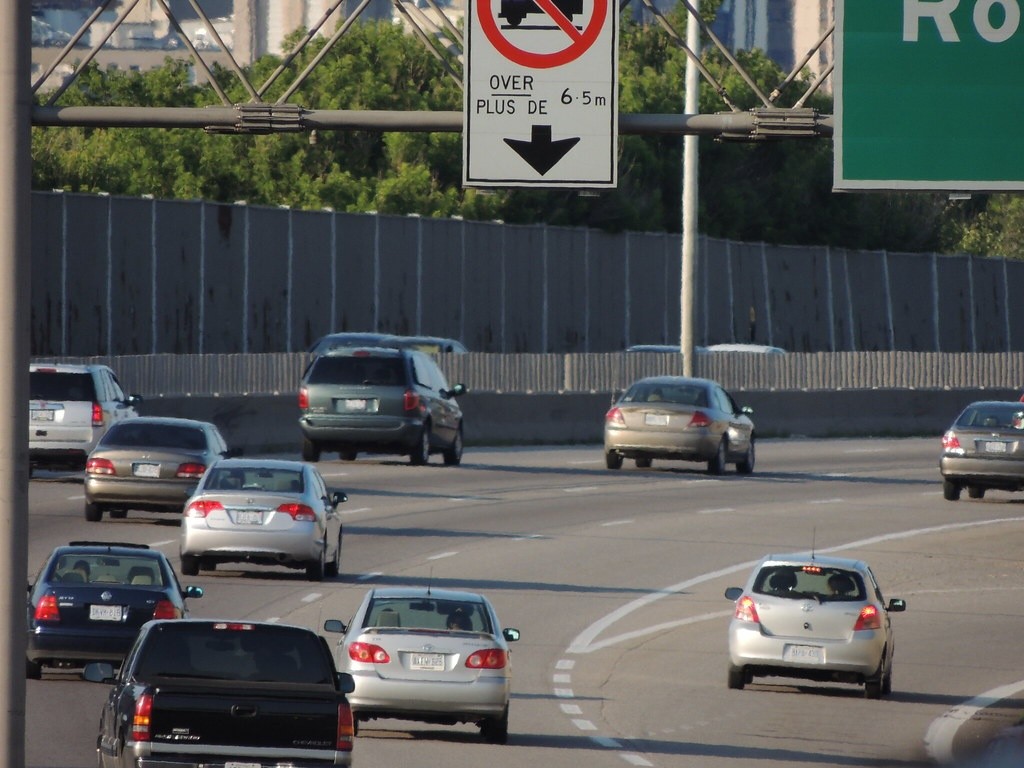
top-left (983, 417), bottom-right (999, 426)
top-left (220, 477), bottom-right (243, 490)
top-left (62, 569), bottom-right (88, 583)
top-left (648, 393), bottom-right (663, 403)
top-left (766, 574), bottom-right (795, 598)
top-left (823, 574), bottom-right (858, 601)
top-left (274, 479), bottom-right (299, 493)
top-left (376, 612), bottom-right (401, 627)
top-left (130, 575), bottom-right (153, 585)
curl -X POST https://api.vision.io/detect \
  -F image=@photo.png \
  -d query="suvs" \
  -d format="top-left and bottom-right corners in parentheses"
top-left (27, 359), bottom-right (146, 482)
top-left (297, 346), bottom-right (469, 465)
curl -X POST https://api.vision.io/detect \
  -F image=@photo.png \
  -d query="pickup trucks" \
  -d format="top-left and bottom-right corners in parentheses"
top-left (78, 617), bottom-right (362, 768)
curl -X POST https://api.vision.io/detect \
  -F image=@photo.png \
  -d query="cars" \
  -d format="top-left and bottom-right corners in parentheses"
top-left (324, 586), bottom-right (520, 744)
top-left (939, 401), bottom-right (1023, 507)
top-left (21, 537), bottom-right (206, 684)
top-left (624, 343), bottom-right (707, 354)
top-left (600, 376), bottom-right (759, 476)
top-left (305, 333), bottom-right (402, 351)
top-left (81, 414), bottom-right (245, 523)
top-left (179, 456), bottom-right (349, 579)
top-left (706, 341), bottom-right (787, 355)
top-left (723, 553), bottom-right (907, 701)
top-left (377, 334), bottom-right (472, 353)
